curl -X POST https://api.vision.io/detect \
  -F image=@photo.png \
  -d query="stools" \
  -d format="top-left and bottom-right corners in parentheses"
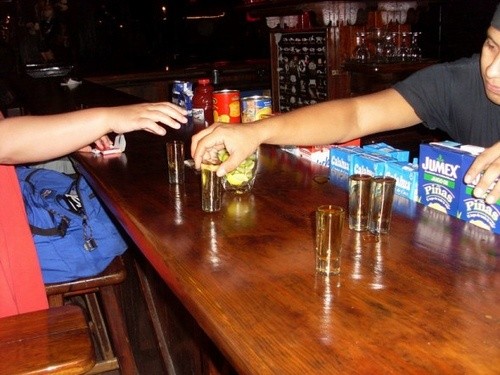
top-left (0, 254), bottom-right (141, 375)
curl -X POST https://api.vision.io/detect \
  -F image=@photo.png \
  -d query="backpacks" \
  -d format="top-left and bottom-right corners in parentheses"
top-left (16, 166), bottom-right (129, 285)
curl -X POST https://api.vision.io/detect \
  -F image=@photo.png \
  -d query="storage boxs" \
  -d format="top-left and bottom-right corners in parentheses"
top-left (290, 137), bottom-right (361, 169)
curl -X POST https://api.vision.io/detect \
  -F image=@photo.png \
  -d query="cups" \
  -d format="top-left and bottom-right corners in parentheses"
top-left (349, 174), bottom-right (372, 231)
top-left (166, 140), bottom-right (185, 184)
top-left (368, 176), bottom-right (397, 234)
top-left (316, 205), bottom-right (343, 274)
top-left (200, 160), bottom-right (222, 212)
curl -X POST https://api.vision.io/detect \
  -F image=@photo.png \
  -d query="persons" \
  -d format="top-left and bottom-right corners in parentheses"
top-left (1, 101), bottom-right (187, 278)
top-left (190, 6), bottom-right (500, 209)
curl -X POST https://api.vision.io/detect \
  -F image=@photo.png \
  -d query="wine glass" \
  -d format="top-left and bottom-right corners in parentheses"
top-left (352, 32), bottom-right (421, 64)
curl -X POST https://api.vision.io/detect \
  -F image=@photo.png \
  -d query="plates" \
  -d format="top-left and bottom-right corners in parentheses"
top-left (25, 64), bottom-right (75, 77)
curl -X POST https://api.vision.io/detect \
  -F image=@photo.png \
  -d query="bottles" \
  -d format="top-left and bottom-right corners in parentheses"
top-left (192, 79), bottom-right (215, 125)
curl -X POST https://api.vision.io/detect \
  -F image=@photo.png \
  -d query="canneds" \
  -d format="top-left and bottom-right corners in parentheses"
top-left (171, 79), bottom-right (272, 125)
